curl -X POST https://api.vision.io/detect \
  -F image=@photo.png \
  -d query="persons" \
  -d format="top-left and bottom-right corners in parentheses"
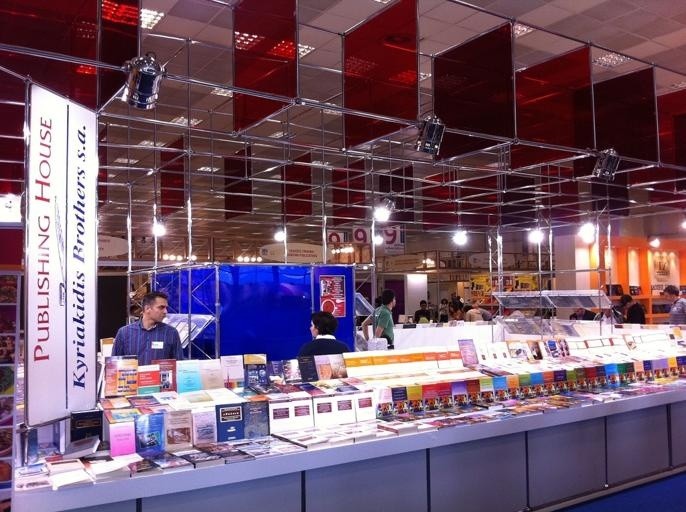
top-left (569, 293), bottom-right (646, 324)
top-left (299, 311), bottom-right (350, 357)
top-left (361, 289), bottom-right (397, 350)
top-left (130, 305), bottom-right (143, 321)
top-left (110, 290), bottom-right (184, 366)
top-left (413, 295), bottom-right (493, 324)
top-left (663, 285), bottom-right (686, 325)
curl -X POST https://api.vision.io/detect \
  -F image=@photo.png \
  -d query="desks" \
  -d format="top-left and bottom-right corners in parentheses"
top-left (9, 369), bottom-right (686, 511)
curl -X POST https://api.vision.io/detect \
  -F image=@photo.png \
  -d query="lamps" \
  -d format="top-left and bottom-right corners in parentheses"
top-left (120, 50), bottom-right (170, 111)
top-left (586, 146), bottom-right (622, 184)
top-left (411, 113), bottom-right (447, 157)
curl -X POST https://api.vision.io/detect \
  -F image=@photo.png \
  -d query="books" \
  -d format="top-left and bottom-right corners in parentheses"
top-left (12, 329), bottom-right (686, 496)
